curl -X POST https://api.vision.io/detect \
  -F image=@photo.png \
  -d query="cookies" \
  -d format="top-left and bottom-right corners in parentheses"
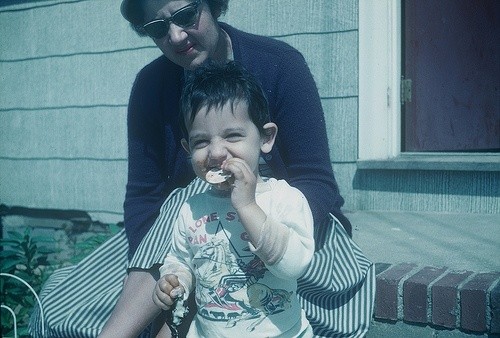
top-left (205, 167), bottom-right (233, 184)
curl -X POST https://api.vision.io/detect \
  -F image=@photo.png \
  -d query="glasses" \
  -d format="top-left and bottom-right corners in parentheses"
top-left (141, 0), bottom-right (202, 39)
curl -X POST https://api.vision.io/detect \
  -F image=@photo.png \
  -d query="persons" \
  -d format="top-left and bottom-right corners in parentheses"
top-left (153, 58), bottom-right (316, 338)
top-left (27, 0), bottom-right (376, 338)
